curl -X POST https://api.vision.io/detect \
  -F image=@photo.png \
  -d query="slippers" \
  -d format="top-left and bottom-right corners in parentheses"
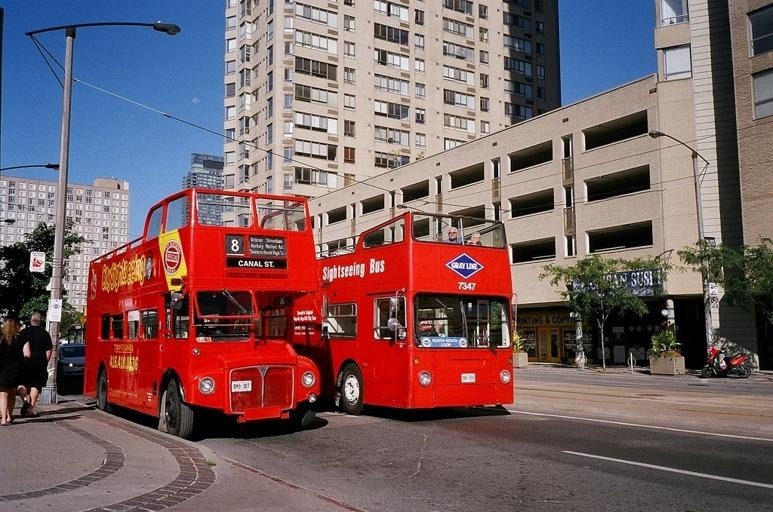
top-left (0, 400), bottom-right (34, 426)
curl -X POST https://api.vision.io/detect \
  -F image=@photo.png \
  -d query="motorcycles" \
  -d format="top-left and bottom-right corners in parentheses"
top-left (703, 347), bottom-right (751, 378)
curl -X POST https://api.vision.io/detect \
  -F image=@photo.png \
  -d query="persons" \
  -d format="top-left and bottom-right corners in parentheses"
top-left (14, 311), bottom-right (53, 416)
top-left (0, 318), bottom-right (20, 425)
top-left (466, 232), bottom-right (482, 246)
top-left (443, 227), bottom-right (461, 244)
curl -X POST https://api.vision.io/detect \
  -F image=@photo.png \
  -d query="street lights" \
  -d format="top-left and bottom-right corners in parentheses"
top-left (23, 21), bottom-right (183, 407)
top-left (646, 130), bottom-right (713, 377)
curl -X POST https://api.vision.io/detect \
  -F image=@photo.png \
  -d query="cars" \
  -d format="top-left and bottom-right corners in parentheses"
top-left (56, 343), bottom-right (85, 380)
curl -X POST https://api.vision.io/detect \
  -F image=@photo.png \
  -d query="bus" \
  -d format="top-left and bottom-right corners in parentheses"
top-left (79, 188), bottom-right (333, 441)
top-left (317, 210), bottom-right (518, 416)
